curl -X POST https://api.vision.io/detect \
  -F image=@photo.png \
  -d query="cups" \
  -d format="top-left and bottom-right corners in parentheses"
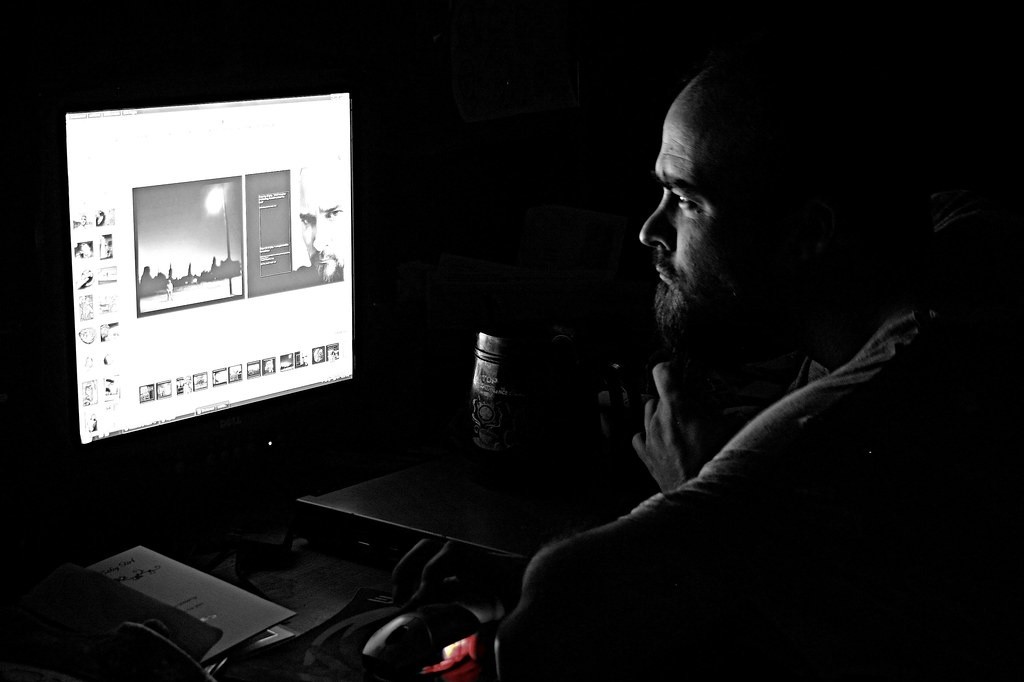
top-left (468, 331), bottom-right (516, 451)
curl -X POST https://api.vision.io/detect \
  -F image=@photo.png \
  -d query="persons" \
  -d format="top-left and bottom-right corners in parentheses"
top-left (494, 56), bottom-right (1024, 682)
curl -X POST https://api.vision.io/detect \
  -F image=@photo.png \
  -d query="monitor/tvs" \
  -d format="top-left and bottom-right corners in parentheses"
top-left (65, 92), bottom-right (357, 463)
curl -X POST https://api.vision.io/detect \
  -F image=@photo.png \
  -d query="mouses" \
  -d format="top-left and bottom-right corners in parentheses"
top-left (361, 603), bottom-right (481, 682)
